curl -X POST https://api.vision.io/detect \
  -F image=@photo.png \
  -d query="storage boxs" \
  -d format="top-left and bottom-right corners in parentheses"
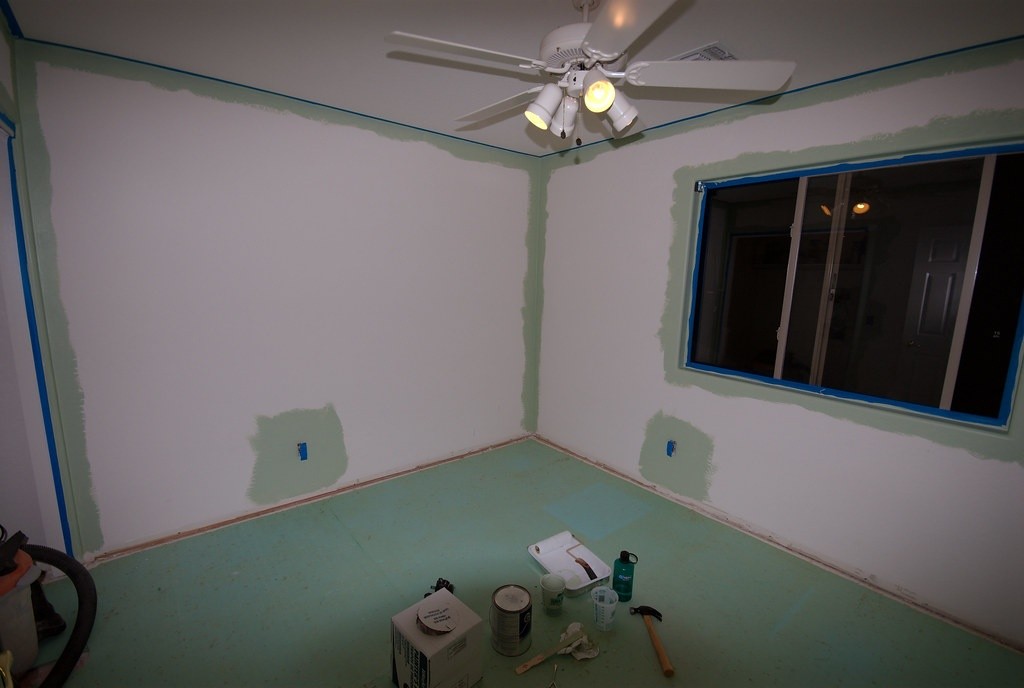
top-left (390, 587), bottom-right (484, 688)
top-left (527, 529), bottom-right (612, 598)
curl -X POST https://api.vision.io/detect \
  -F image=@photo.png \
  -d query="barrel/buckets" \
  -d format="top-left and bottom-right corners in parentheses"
top-left (491, 584), bottom-right (532, 656)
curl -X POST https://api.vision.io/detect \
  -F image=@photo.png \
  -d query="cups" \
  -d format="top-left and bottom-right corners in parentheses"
top-left (540, 573), bottom-right (565, 616)
top-left (591, 586), bottom-right (619, 631)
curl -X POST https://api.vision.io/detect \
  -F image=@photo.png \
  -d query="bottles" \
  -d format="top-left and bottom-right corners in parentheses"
top-left (613, 551), bottom-right (634, 602)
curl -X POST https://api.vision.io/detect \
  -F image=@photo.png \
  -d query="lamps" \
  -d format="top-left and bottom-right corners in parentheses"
top-left (583, 69), bottom-right (616, 114)
top-left (607, 88), bottom-right (638, 132)
top-left (818, 199), bottom-right (833, 217)
top-left (524, 83), bottom-right (563, 131)
top-left (852, 192), bottom-right (872, 215)
top-left (549, 95), bottom-right (580, 138)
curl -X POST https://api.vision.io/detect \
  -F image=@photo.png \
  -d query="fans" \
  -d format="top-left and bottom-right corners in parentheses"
top-left (383, 0), bottom-right (798, 123)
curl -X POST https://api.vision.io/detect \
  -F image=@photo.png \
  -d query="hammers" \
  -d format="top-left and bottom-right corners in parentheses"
top-left (628, 606), bottom-right (673, 678)
